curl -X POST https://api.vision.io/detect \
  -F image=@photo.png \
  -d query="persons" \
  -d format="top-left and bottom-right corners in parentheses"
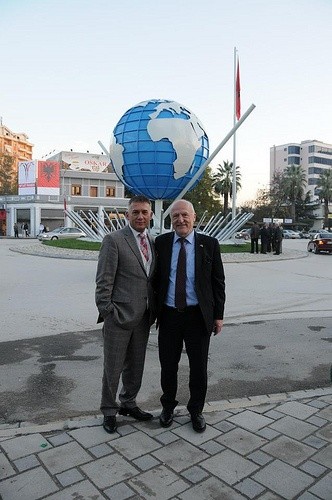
top-left (149, 200), bottom-right (226, 432)
top-left (95, 197), bottom-right (157, 433)
top-left (251, 221), bottom-right (284, 255)
top-left (3, 223), bottom-right (50, 239)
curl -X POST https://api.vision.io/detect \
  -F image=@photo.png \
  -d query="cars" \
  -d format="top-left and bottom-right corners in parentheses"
top-left (307, 233), bottom-right (332, 254)
top-left (283, 229), bottom-right (332, 239)
top-left (38, 227), bottom-right (87, 241)
top-left (235, 229), bottom-right (252, 240)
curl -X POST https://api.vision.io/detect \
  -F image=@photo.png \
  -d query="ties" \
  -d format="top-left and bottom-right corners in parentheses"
top-left (175, 238), bottom-right (187, 314)
top-left (137, 231), bottom-right (152, 271)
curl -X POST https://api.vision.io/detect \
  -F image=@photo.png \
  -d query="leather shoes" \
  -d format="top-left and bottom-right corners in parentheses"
top-left (119, 405), bottom-right (154, 421)
top-left (103, 414), bottom-right (118, 434)
top-left (188, 407), bottom-right (206, 433)
top-left (159, 408), bottom-right (174, 428)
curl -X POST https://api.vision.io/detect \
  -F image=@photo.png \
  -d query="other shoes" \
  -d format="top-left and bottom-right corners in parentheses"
top-left (263, 252), bottom-right (267, 254)
top-left (267, 251), bottom-right (271, 252)
top-left (250, 252), bottom-right (254, 253)
top-left (256, 252), bottom-right (258, 254)
top-left (260, 252), bottom-right (263, 254)
top-left (273, 253), bottom-right (279, 255)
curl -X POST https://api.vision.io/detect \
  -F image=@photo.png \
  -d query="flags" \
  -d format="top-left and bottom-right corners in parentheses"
top-left (236, 54), bottom-right (241, 120)
